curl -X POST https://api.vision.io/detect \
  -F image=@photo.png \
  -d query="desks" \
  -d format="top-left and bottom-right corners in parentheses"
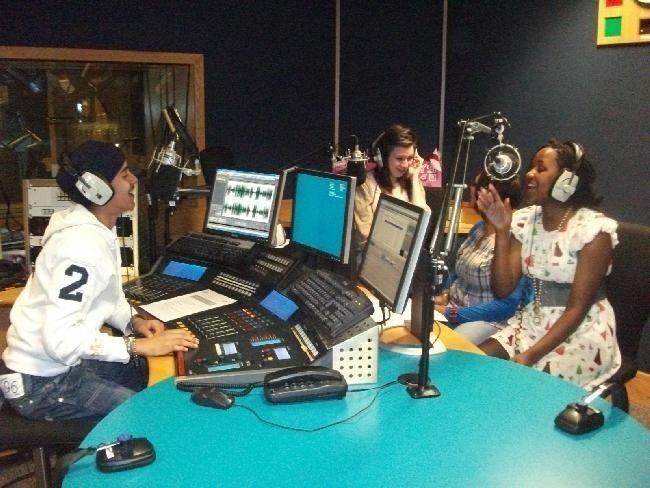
top-left (2, 263), bottom-right (483, 388)
top-left (61, 346), bottom-right (650, 488)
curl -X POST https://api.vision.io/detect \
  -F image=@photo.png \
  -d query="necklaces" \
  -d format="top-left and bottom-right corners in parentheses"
top-left (531, 204), bottom-right (576, 326)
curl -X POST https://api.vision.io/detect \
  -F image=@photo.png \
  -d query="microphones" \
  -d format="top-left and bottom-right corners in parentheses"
top-left (484, 116), bottom-right (520, 180)
top-left (348, 136), bottom-right (370, 187)
top-left (147, 133), bottom-right (180, 201)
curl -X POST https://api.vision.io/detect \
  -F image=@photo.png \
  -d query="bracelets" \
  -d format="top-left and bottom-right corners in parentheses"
top-left (132, 337), bottom-right (138, 360)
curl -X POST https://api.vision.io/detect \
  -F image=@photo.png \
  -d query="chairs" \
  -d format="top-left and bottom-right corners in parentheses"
top-left (504, 222), bottom-right (649, 411)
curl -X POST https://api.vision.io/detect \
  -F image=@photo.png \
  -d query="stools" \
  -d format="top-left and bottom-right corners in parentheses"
top-left (0, 375), bottom-right (104, 488)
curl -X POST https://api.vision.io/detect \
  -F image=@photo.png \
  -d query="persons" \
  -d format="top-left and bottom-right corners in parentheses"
top-left (0, 139), bottom-right (199, 423)
top-left (433, 169), bottom-right (527, 345)
top-left (353, 124), bottom-right (427, 247)
top-left (477, 137), bottom-right (622, 392)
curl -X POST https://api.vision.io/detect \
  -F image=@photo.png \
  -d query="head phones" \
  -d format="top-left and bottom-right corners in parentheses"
top-left (55, 151), bottom-right (114, 208)
top-left (550, 142), bottom-right (586, 202)
top-left (371, 130), bottom-right (420, 169)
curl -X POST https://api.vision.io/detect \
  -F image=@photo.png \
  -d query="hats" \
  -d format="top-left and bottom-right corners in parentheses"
top-left (56, 140), bottom-right (126, 208)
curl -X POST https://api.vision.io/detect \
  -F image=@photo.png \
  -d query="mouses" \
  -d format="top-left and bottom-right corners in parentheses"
top-left (193, 386), bottom-right (235, 410)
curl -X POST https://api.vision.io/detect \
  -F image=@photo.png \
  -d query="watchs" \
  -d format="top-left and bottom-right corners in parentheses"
top-left (123, 335), bottom-right (133, 358)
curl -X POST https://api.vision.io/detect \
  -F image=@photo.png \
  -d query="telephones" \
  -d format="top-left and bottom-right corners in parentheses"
top-left (176, 366), bottom-right (349, 405)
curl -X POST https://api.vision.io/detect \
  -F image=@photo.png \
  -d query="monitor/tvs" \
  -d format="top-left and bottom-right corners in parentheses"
top-left (289, 167), bottom-right (357, 267)
top-left (201, 167), bottom-right (286, 247)
top-left (357, 192), bottom-right (431, 314)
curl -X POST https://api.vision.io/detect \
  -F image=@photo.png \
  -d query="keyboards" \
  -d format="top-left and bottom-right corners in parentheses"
top-left (246, 249), bottom-right (302, 287)
top-left (166, 230), bottom-right (256, 267)
top-left (287, 270), bottom-right (373, 338)
